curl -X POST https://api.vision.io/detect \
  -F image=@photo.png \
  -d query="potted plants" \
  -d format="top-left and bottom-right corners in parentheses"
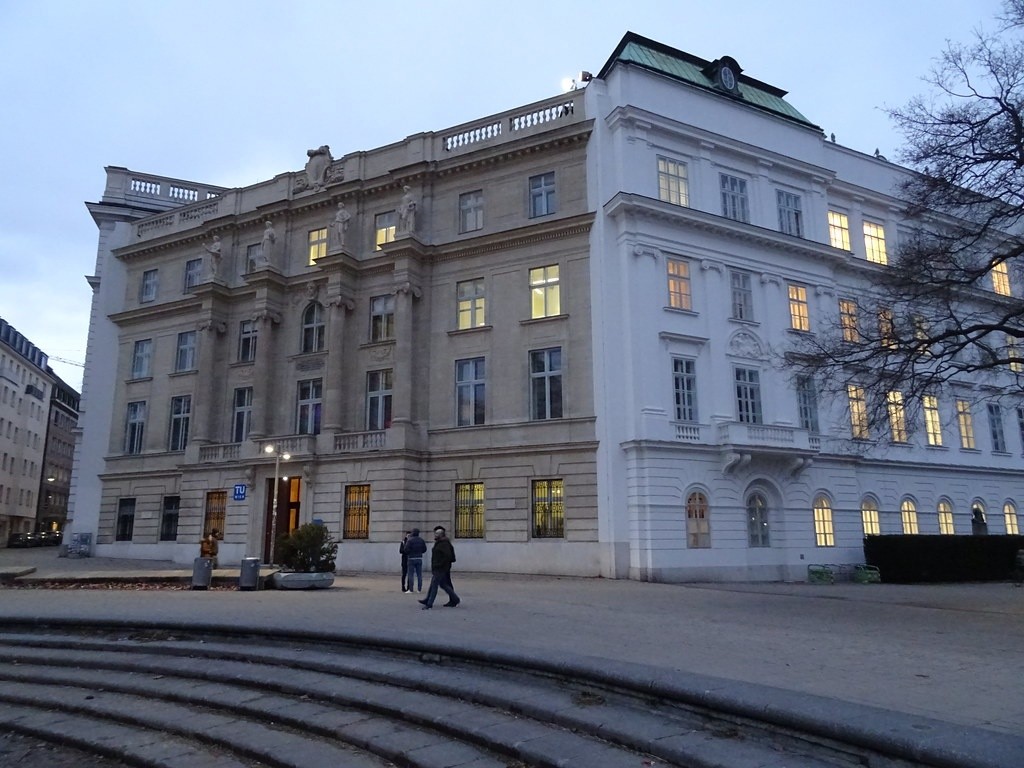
top-left (273, 522), bottom-right (338, 591)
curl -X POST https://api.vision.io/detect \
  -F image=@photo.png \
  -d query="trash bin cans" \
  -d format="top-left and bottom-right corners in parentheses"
top-left (190, 557), bottom-right (213, 591)
top-left (239, 556), bottom-right (261, 590)
top-left (58, 544), bottom-right (69, 558)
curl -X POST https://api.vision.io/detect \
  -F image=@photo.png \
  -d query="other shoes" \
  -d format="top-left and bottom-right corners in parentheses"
top-left (402, 587), bottom-right (406, 591)
top-left (418, 599), bottom-right (427, 604)
top-left (417, 591), bottom-right (420, 593)
top-left (444, 600), bottom-right (460, 607)
top-left (405, 590), bottom-right (412, 593)
top-left (423, 606), bottom-right (433, 610)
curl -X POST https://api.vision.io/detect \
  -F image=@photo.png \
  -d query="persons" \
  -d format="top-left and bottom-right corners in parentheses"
top-left (418, 525), bottom-right (455, 607)
top-left (261, 221), bottom-right (275, 261)
top-left (202, 235), bottom-right (223, 278)
top-left (200, 533), bottom-right (212, 558)
top-left (399, 531), bottom-right (409, 592)
top-left (405, 529), bottom-right (427, 593)
top-left (395, 187), bottom-right (415, 231)
top-left (327, 202), bottom-right (351, 248)
top-left (421, 529), bottom-right (461, 610)
top-left (210, 528), bottom-right (219, 570)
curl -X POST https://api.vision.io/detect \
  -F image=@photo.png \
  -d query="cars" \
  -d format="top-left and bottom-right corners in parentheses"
top-left (7, 530), bottom-right (61, 548)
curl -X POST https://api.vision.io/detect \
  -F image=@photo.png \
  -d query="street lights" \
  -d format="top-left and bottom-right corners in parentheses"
top-left (265, 445), bottom-right (290, 569)
